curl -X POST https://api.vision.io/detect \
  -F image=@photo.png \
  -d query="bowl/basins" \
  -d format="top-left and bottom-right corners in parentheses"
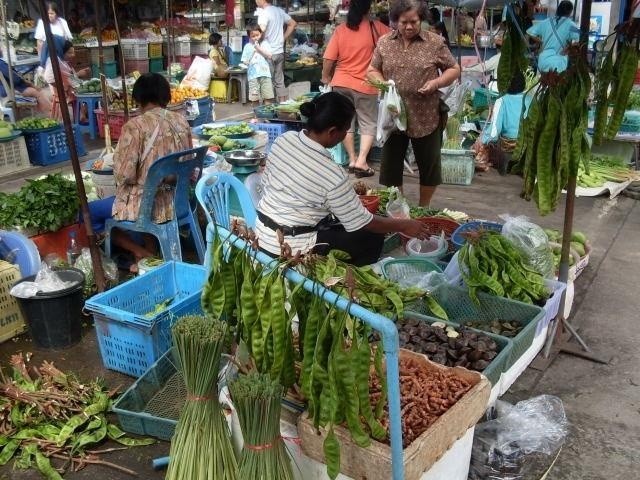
top-left (405, 236), bottom-right (450, 262)
top-left (222, 149), bottom-right (268, 166)
top-left (360, 193), bottom-right (380, 216)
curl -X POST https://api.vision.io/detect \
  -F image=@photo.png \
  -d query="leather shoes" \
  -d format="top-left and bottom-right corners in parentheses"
top-left (348, 165), bottom-right (376, 178)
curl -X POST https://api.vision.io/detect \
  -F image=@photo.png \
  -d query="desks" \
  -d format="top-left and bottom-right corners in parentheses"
top-left (225, 69), bottom-right (249, 105)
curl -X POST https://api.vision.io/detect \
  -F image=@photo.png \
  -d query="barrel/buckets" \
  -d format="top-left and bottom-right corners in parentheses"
top-left (477, 35), bottom-right (495, 49)
top-left (90, 173), bottom-right (116, 200)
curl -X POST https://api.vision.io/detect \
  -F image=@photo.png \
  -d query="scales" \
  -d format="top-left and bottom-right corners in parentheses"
top-left (223, 150), bottom-right (266, 217)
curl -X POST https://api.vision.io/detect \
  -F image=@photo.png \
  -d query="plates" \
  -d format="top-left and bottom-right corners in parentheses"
top-left (192, 121), bottom-right (260, 139)
top-left (86, 159), bottom-right (113, 175)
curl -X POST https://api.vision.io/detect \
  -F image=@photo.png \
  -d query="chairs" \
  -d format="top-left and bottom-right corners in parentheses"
top-left (194, 169), bottom-right (257, 235)
top-left (101, 142), bottom-right (210, 269)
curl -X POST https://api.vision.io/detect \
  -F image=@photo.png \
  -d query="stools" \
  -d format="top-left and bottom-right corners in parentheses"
top-left (496, 149), bottom-right (519, 177)
top-left (72, 91), bottom-right (104, 142)
top-left (0, 107), bottom-right (16, 123)
top-left (209, 76), bottom-right (238, 104)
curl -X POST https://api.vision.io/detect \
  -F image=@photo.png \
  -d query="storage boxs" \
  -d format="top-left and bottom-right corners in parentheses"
top-left (534, 278), bottom-right (567, 338)
top-left (70, 46), bottom-right (118, 80)
top-left (295, 346), bottom-right (493, 479)
top-left (149, 54), bottom-right (209, 73)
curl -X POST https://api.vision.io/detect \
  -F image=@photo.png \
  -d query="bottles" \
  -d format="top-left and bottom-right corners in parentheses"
top-left (67, 231), bottom-right (80, 267)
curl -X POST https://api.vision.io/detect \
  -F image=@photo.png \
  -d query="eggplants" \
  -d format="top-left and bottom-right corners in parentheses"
top-left (545, 229), bottom-right (586, 268)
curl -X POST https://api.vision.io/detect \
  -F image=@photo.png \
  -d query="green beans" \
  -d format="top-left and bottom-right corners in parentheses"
top-left (0, 367), bottom-right (158, 480)
top-left (496, 29), bottom-right (640, 216)
top-left (457, 229), bottom-right (550, 307)
top-left (201, 222), bottom-right (449, 480)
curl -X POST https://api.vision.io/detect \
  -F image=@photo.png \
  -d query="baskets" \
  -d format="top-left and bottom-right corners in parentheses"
top-left (440, 155), bottom-right (475, 185)
top-left (84, 260), bottom-right (210, 378)
top-left (358, 195), bottom-right (379, 214)
top-left (381, 217), bottom-right (504, 254)
top-left (0, 259), bottom-right (29, 343)
top-left (391, 273), bottom-right (568, 389)
top-left (473, 87), bottom-right (500, 109)
top-left (381, 256), bottom-right (444, 283)
top-left (67, 35), bottom-right (242, 79)
top-left (24, 124), bottom-right (87, 166)
top-left (0, 136), bottom-right (33, 178)
top-left (93, 108), bottom-right (141, 140)
top-left (253, 123), bottom-right (287, 152)
top-left (169, 98), bottom-right (215, 128)
top-left (111, 346), bottom-right (210, 442)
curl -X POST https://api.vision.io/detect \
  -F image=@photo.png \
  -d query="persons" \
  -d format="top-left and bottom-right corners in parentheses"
top-left (425, 8), bottom-right (451, 52)
top-left (366, 0), bottom-right (460, 205)
top-left (34, 2), bottom-right (74, 69)
top-left (240, 23), bottom-right (272, 105)
top-left (0, 45), bottom-right (49, 112)
top-left (78, 72), bottom-right (199, 273)
top-left (255, 91), bottom-right (432, 267)
top-left (43, 40), bottom-right (90, 124)
top-left (465, 35), bottom-right (504, 92)
top-left (253, 0), bottom-right (297, 105)
top-left (208, 32), bottom-right (230, 77)
top-left (483, 72), bottom-right (534, 146)
top-left (526, 0), bottom-right (580, 75)
top-left (320, 0), bottom-right (391, 172)
